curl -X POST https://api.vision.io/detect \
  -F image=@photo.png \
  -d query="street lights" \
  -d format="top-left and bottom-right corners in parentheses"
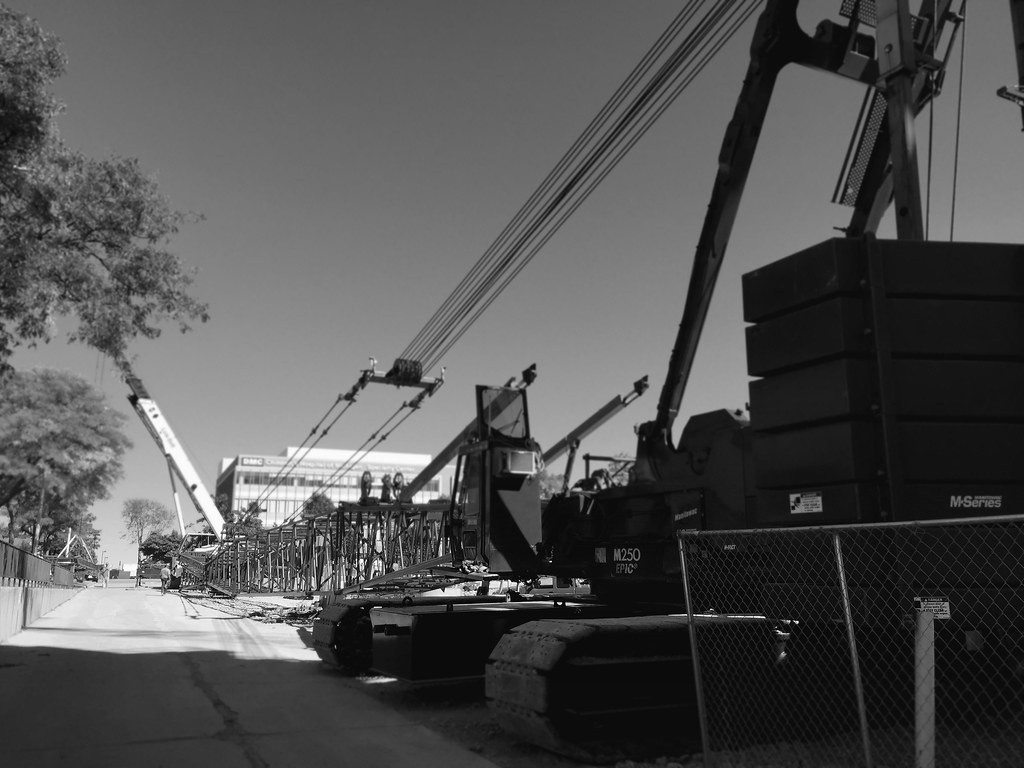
top-left (101, 551), bottom-right (108, 564)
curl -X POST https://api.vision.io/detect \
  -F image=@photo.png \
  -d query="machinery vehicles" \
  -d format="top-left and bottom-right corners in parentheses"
top-left (112, 345), bottom-right (228, 590)
top-left (296, 1), bottom-right (1024, 768)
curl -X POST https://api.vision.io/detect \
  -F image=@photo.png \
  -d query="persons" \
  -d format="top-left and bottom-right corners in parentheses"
top-left (160, 565), bottom-right (171, 596)
